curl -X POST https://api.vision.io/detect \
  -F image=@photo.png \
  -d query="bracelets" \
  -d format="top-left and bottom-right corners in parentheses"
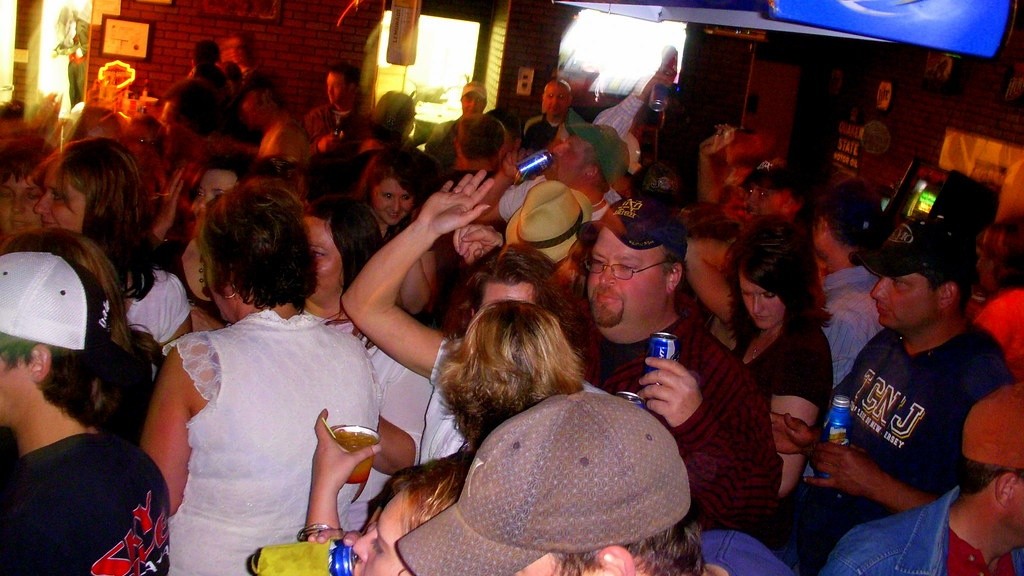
top-left (297, 523), bottom-right (343, 542)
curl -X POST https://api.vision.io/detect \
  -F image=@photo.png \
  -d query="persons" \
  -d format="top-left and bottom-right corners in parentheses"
top-left (0, 41), bottom-right (1024, 576)
top-left (0, 250), bottom-right (169, 576)
top-left (818, 380), bottom-right (1024, 576)
top-left (396, 391), bottom-right (796, 576)
top-left (138, 182), bottom-right (380, 575)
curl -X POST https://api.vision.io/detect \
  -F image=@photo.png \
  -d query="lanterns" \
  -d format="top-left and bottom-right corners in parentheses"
top-left (875, 79), bottom-right (893, 112)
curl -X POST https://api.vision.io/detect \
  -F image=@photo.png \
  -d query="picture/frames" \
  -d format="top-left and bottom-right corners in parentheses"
top-left (192, 0), bottom-right (283, 27)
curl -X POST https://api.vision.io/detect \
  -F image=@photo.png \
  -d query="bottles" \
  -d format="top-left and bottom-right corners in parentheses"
top-left (661, 52), bottom-right (678, 88)
top-left (819, 395), bottom-right (851, 479)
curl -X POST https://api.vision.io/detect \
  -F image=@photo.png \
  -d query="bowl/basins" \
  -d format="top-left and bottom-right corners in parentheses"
top-left (140, 96), bottom-right (159, 106)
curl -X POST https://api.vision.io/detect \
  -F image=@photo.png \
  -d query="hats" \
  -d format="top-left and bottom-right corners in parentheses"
top-left (577, 196), bottom-right (689, 253)
top-left (740, 159), bottom-right (800, 190)
top-left (850, 215), bottom-right (979, 278)
top-left (461, 80), bottom-right (488, 101)
top-left (505, 180), bottom-right (591, 266)
top-left (566, 107), bottom-right (630, 185)
top-left (963, 383), bottom-right (1024, 467)
top-left (0, 252), bottom-right (110, 352)
top-left (395, 390), bottom-right (690, 576)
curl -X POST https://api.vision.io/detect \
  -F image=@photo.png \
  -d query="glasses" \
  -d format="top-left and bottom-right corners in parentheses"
top-left (584, 257), bottom-right (673, 281)
top-left (744, 188), bottom-right (770, 200)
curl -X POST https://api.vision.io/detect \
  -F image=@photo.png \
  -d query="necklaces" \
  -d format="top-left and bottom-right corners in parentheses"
top-left (748, 331), bottom-right (775, 359)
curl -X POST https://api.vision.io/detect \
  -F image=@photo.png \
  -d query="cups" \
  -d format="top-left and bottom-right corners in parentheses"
top-left (332, 424), bottom-right (381, 483)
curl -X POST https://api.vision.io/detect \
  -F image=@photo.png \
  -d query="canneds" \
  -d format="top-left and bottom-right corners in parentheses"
top-left (642, 331), bottom-right (679, 385)
top-left (616, 391), bottom-right (643, 408)
top-left (328, 538), bottom-right (358, 576)
top-left (515, 148), bottom-right (553, 178)
top-left (648, 83), bottom-right (670, 111)
